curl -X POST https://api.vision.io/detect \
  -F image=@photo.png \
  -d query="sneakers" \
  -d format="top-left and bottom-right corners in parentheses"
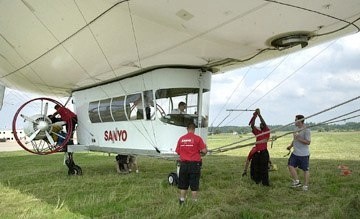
top-left (302, 185), bottom-right (308, 191)
top-left (292, 180), bottom-right (302, 187)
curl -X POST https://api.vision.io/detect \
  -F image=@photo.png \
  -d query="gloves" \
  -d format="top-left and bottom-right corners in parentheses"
top-left (242, 170), bottom-right (247, 178)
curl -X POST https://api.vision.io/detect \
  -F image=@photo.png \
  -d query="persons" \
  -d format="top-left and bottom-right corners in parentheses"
top-left (287, 115), bottom-right (311, 190)
top-left (242, 147), bottom-right (272, 179)
top-left (134, 93), bottom-right (154, 120)
top-left (51, 104), bottom-right (77, 145)
top-left (250, 109), bottom-right (270, 187)
top-left (173, 102), bottom-right (187, 114)
top-left (175, 122), bottom-right (207, 206)
